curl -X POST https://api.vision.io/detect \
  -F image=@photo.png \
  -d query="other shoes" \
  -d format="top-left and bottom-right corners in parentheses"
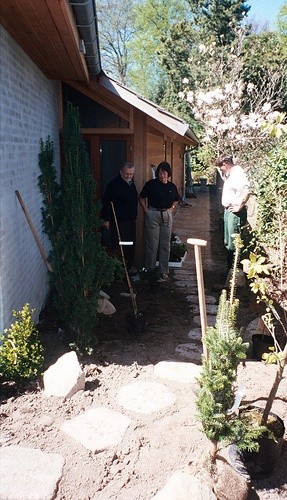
top-left (162, 274), bottom-right (168, 279)
top-left (129, 269), bottom-right (136, 276)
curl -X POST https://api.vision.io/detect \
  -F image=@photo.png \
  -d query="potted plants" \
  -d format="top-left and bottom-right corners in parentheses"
top-left (168, 243), bottom-right (188, 267)
top-left (228, 251), bottom-right (287, 480)
top-left (150, 266), bottom-right (162, 294)
top-left (193, 289), bottom-right (250, 429)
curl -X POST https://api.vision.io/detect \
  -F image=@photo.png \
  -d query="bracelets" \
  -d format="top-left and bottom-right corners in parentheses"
top-left (171, 205), bottom-right (176, 210)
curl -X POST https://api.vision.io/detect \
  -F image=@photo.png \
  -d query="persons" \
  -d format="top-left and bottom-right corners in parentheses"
top-left (138, 162), bottom-right (180, 279)
top-left (100, 160), bottom-right (138, 298)
top-left (213, 154), bottom-right (251, 272)
